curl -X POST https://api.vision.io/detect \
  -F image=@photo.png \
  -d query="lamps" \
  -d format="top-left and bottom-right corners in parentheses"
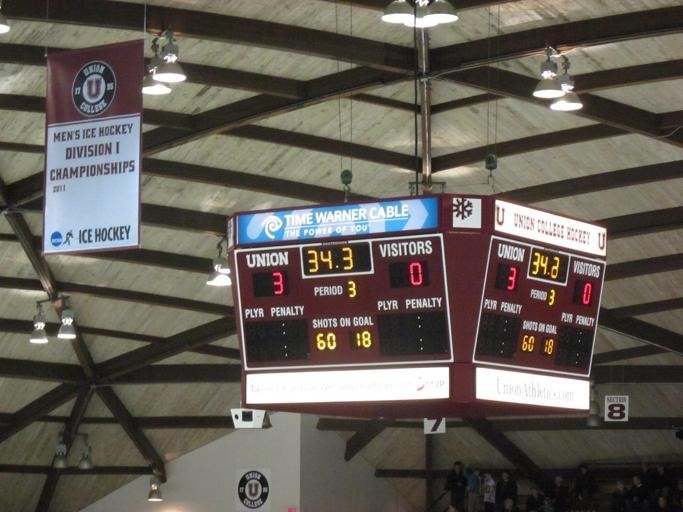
top-left (27, 294), bottom-right (80, 342)
top-left (145, 472), bottom-right (165, 503)
top-left (528, 40), bottom-right (587, 114)
top-left (50, 429), bottom-right (93, 472)
top-left (139, 18), bottom-right (188, 96)
top-left (378, 1), bottom-right (461, 31)
top-left (203, 232), bottom-right (231, 289)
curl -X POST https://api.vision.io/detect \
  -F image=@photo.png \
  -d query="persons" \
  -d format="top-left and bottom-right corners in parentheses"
top-left (444, 460), bottom-right (683, 512)
